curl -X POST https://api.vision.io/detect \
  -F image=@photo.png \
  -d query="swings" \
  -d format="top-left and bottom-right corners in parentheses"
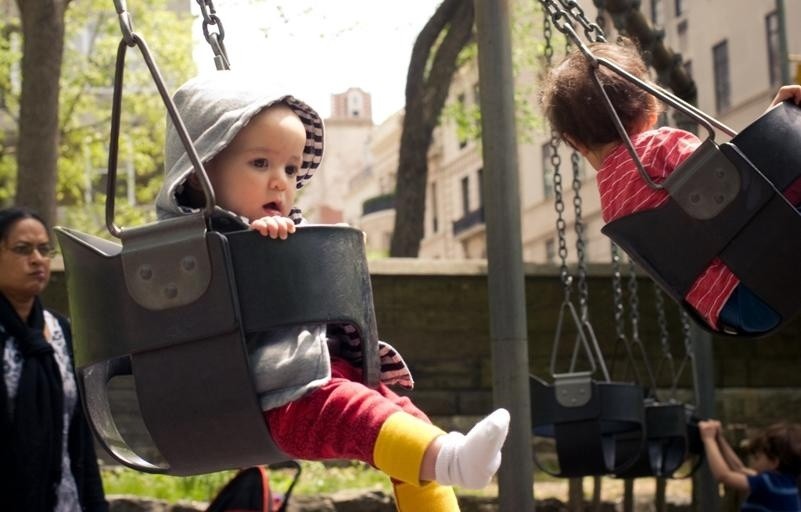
top-left (54, 0), bottom-right (382, 476)
top-left (529, 2), bottom-right (710, 477)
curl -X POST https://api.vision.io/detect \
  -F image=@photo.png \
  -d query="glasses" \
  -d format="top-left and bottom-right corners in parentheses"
top-left (4, 242), bottom-right (52, 255)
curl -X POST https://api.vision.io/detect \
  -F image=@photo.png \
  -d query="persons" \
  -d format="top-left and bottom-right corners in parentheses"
top-left (152, 67), bottom-right (515, 511)
top-left (0, 207), bottom-right (112, 511)
top-left (695, 411), bottom-right (801, 510)
